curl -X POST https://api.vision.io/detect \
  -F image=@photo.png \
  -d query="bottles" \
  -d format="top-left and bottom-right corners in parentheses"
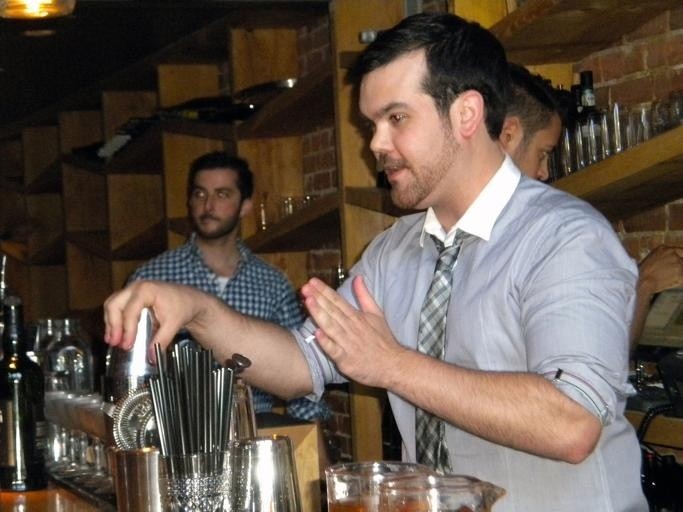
top-left (575, 70), bottom-right (603, 171)
top-left (258, 203), bottom-right (270, 234)
top-left (44, 314), bottom-right (95, 396)
top-left (0, 296), bottom-right (47, 494)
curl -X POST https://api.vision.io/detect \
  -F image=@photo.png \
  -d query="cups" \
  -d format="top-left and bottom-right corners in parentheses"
top-left (160, 452), bottom-right (234, 512)
top-left (236, 437), bottom-right (303, 512)
top-left (278, 194), bottom-right (316, 217)
top-left (377, 473), bottom-right (506, 512)
top-left (108, 446), bottom-right (161, 512)
top-left (325, 460), bottom-right (431, 512)
top-left (555, 102), bottom-right (654, 177)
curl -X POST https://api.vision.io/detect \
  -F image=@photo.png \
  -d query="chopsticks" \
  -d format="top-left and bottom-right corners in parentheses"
top-left (149, 341), bottom-right (233, 453)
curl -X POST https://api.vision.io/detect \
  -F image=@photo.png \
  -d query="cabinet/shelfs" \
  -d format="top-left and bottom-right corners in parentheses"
top-left (0, 1), bottom-right (682, 464)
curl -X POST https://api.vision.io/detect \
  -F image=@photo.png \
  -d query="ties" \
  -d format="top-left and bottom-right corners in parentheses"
top-left (416, 228), bottom-right (470, 475)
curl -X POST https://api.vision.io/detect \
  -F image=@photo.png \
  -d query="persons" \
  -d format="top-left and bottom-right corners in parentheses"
top-left (120, 150), bottom-right (332, 428)
top-left (105, 10), bottom-right (648, 512)
top-left (501, 60), bottom-right (681, 351)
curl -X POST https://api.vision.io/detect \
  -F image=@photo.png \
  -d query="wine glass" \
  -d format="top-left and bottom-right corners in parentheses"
top-left (34, 390), bottom-right (123, 496)
